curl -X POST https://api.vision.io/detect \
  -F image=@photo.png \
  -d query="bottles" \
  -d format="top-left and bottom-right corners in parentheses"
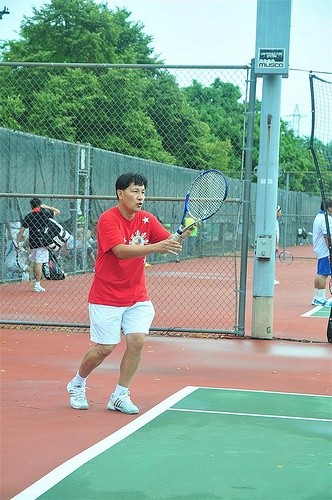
top-left (26, 272), bottom-right (29, 282)
top-left (22, 272), bottom-right (26, 282)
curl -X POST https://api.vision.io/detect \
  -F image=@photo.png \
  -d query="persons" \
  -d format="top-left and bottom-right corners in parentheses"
top-left (313, 197), bottom-right (332, 307)
top-left (187, 226), bottom-right (198, 258)
top-left (274, 205), bottom-right (282, 284)
top-left (15, 198), bottom-right (60, 292)
top-left (66, 173), bottom-right (197, 413)
top-left (64, 229), bottom-right (96, 273)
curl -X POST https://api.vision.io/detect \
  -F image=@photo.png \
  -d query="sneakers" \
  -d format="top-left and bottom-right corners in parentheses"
top-left (312, 297), bottom-right (315, 304)
top-left (33, 285), bottom-right (46, 293)
top-left (67, 377), bottom-right (90, 411)
top-left (108, 391), bottom-right (140, 414)
top-left (315, 298), bottom-right (332, 307)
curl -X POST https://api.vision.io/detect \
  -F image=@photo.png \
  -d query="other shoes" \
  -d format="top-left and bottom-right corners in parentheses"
top-left (274, 279), bottom-right (280, 285)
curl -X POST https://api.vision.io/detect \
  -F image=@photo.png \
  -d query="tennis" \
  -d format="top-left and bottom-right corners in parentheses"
top-left (184, 218), bottom-right (195, 228)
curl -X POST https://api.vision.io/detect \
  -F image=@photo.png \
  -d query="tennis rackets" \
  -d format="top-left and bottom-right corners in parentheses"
top-left (276, 247), bottom-right (294, 266)
top-left (11, 239), bottom-right (30, 268)
top-left (161, 169), bottom-right (229, 257)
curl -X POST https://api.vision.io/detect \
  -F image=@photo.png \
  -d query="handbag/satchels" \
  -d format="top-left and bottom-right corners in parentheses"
top-left (42, 217), bottom-right (73, 252)
